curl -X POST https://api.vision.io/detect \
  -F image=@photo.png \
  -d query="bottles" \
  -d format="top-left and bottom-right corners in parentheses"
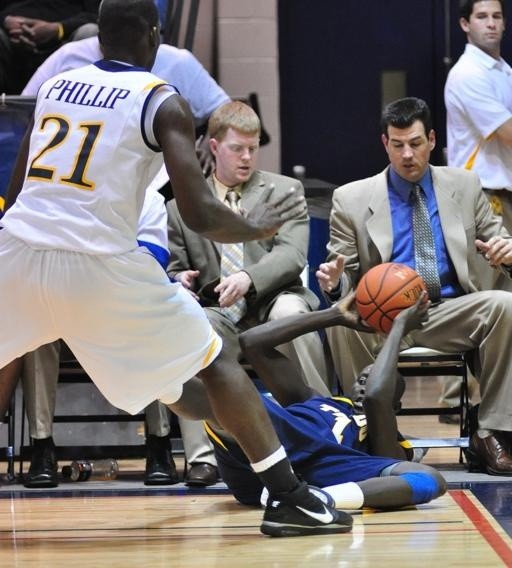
top-left (292, 164), bottom-right (307, 181)
top-left (60, 458), bottom-right (119, 482)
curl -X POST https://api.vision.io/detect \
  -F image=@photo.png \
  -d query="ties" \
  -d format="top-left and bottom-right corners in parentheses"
top-left (219, 192), bottom-right (249, 326)
top-left (409, 185), bottom-right (441, 302)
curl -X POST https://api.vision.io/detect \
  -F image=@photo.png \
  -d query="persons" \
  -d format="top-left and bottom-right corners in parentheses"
top-left (0, 199), bottom-right (25, 483)
top-left (169, 102), bottom-right (329, 484)
top-left (21, 1), bottom-right (232, 126)
top-left (438, 0), bottom-right (511, 425)
top-left (315, 98), bottom-right (510, 476)
top-left (162, 289), bottom-right (449, 513)
top-left (2, 2), bottom-right (353, 535)
top-left (24, 185), bottom-right (176, 489)
top-left (3, 1), bottom-right (90, 98)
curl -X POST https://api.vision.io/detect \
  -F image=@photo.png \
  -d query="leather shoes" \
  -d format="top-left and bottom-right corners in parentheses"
top-left (184, 461), bottom-right (220, 486)
top-left (464, 431), bottom-right (512, 476)
top-left (143, 438), bottom-right (180, 484)
top-left (27, 441), bottom-right (58, 487)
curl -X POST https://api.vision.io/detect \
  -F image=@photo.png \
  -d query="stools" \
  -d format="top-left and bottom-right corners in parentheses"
top-left (379, 346), bottom-right (476, 468)
top-left (20, 344), bottom-right (154, 479)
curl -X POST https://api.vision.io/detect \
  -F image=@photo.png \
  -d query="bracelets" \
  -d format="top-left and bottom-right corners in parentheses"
top-left (58, 23), bottom-right (64, 41)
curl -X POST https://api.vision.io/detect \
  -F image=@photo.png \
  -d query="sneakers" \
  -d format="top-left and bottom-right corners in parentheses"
top-left (260, 485), bottom-right (353, 536)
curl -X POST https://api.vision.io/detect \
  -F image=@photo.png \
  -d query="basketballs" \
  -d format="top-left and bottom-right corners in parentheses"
top-left (356, 263), bottom-right (428, 333)
